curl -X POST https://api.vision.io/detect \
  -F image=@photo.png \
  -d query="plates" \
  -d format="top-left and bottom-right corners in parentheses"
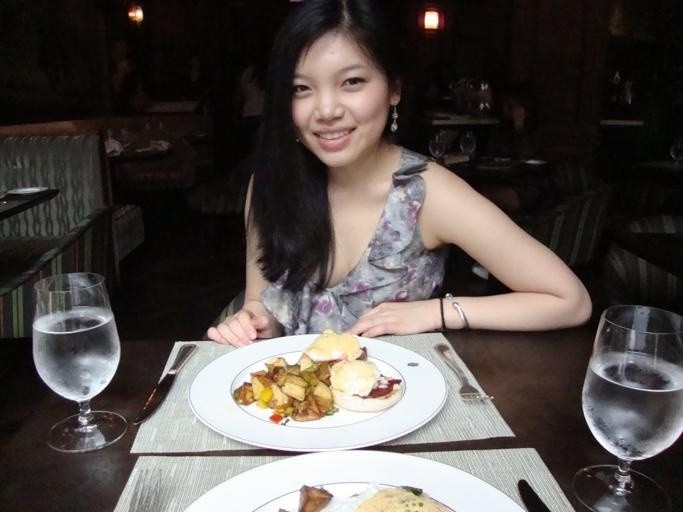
top-left (8, 186), bottom-right (49, 195)
top-left (183, 450), bottom-right (525, 512)
top-left (187, 333), bottom-right (448, 453)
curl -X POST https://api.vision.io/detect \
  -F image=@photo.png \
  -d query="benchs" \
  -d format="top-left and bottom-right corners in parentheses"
top-left (0, 136), bottom-right (100, 340)
top-left (0, 122), bottom-right (146, 290)
top-left (100, 113), bottom-right (212, 191)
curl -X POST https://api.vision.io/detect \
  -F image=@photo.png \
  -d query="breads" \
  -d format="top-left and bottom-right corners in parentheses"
top-left (333, 359), bottom-right (402, 411)
top-left (308, 329), bottom-right (369, 364)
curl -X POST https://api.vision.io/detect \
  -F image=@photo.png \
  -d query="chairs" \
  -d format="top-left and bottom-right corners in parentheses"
top-left (595, 214), bottom-right (682, 317)
top-left (488, 185), bottom-right (615, 291)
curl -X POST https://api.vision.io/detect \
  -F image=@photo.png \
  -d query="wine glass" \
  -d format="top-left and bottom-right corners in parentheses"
top-left (572, 304), bottom-right (683, 512)
top-left (31, 272), bottom-right (129, 454)
top-left (669, 140), bottom-right (683, 168)
top-left (430, 128), bottom-right (477, 163)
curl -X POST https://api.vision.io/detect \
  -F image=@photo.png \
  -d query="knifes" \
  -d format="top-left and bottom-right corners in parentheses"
top-left (518, 479), bottom-right (551, 512)
top-left (133, 345), bottom-right (197, 426)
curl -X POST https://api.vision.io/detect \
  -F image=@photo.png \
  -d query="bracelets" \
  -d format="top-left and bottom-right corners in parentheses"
top-left (440, 291), bottom-right (470, 331)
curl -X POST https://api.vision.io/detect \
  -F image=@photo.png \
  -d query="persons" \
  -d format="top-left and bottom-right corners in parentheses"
top-left (238, 55), bottom-right (266, 137)
top-left (207, 1), bottom-right (594, 347)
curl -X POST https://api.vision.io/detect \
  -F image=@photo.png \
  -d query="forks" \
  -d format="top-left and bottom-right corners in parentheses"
top-left (435, 342), bottom-right (494, 402)
